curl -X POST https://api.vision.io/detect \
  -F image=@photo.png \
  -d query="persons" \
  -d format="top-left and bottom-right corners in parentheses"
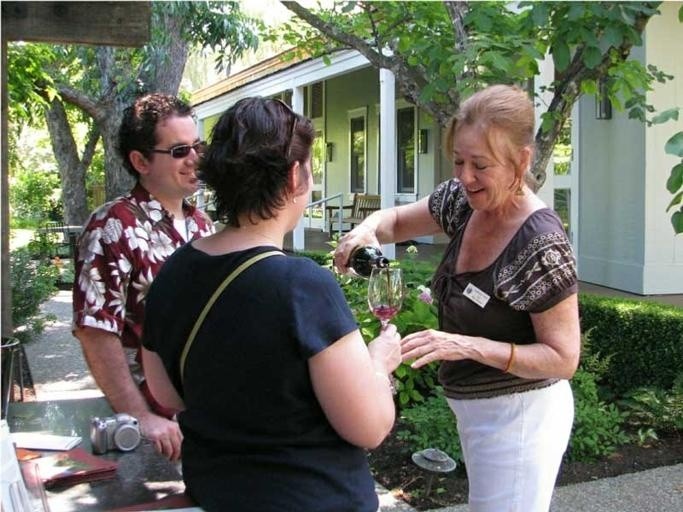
top-left (70, 92), bottom-right (218, 475)
top-left (337, 84), bottom-right (583, 512)
top-left (140, 96), bottom-right (403, 511)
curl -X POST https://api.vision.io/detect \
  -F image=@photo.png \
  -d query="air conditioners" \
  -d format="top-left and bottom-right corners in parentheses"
top-left (324, 143), bottom-right (331, 162)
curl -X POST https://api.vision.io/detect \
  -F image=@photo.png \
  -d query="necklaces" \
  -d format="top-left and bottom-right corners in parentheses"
top-left (229, 221), bottom-right (283, 249)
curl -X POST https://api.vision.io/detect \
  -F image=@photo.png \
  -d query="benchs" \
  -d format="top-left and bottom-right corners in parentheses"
top-left (326, 193), bottom-right (381, 240)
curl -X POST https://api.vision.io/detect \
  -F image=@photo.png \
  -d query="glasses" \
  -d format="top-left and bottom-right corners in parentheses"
top-left (145, 141), bottom-right (201, 159)
top-left (274, 98), bottom-right (298, 167)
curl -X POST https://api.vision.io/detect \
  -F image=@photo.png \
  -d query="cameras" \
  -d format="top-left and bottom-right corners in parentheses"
top-left (89, 413), bottom-right (142, 454)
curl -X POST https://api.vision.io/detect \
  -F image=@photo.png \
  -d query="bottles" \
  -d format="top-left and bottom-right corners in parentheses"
top-left (332, 244), bottom-right (390, 282)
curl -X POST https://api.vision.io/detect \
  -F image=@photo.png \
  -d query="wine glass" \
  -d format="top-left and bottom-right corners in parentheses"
top-left (366, 266), bottom-right (404, 396)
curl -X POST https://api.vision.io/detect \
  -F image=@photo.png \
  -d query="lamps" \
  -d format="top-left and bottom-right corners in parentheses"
top-left (595, 80), bottom-right (612, 119)
top-left (419, 129), bottom-right (428, 154)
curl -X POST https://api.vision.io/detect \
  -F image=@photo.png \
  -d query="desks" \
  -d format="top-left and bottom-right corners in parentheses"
top-left (8, 398), bottom-right (206, 512)
top-left (0, 337), bottom-right (23, 418)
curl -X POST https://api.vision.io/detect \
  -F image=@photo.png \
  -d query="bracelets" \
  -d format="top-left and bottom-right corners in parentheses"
top-left (505, 343), bottom-right (514, 374)
